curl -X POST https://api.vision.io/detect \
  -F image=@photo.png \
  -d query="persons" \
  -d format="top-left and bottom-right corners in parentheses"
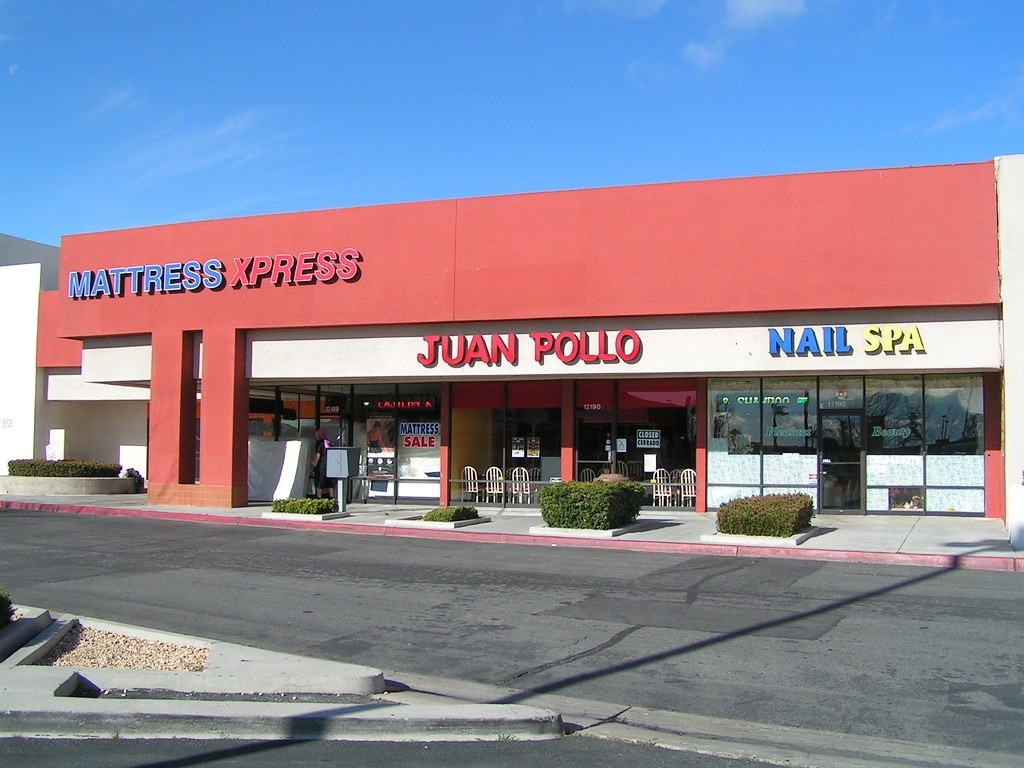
top-left (312, 429), bottom-right (337, 499)
top-left (367, 421), bottom-right (387, 453)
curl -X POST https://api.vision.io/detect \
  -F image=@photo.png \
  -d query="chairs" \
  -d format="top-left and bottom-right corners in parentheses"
top-left (580, 461), bottom-right (644, 483)
top-left (652, 468), bottom-right (697, 508)
top-left (460, 466), bottom-right (541, 505)
